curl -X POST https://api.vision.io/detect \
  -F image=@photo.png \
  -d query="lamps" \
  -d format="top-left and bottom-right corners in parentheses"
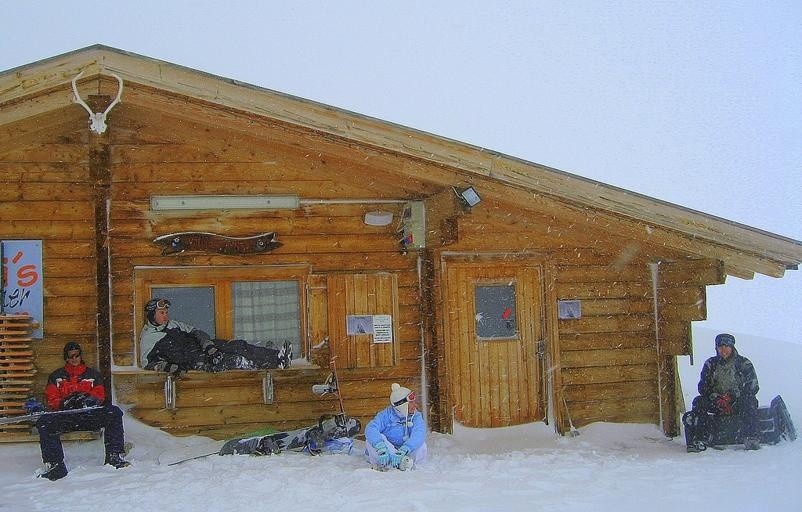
top-left (460, 186), bottom-right (482, 208)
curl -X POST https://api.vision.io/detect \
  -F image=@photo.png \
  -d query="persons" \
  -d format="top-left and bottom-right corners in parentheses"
top-left (364, 382), bottom-right (428, 472)
top-left (682, 333), bottom-right (760, 452)
top-left (36, 341), bottom-right (131, 482)
top-left (140, 298), bottom-right (293, 379)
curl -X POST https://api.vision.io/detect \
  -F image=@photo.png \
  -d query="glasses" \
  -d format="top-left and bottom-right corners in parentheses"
top-left (66, 353), bottom-right (80, 359)
top-left (156, 299), bottom-right (171, 309)
top-left (406, 391), bottom-right (416, 402)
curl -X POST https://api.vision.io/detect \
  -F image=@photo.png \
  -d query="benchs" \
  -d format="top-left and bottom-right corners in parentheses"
top-left (0, 427), bottom-right (105, 443)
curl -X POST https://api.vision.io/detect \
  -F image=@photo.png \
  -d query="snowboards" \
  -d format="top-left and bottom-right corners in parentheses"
top-left (220, 415), bottom-right (361, 455)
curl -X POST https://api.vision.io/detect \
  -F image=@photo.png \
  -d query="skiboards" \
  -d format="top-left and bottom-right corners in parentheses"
top-left (0, 405), bottom-right (105, 425)
top-left (157, 428), bottom-right (280, 466)
top-left (312, 356), bottom-right (349, 439)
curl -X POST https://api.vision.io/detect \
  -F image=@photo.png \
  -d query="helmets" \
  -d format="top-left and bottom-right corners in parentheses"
top-left (145, 298), bottom-right (169, 311)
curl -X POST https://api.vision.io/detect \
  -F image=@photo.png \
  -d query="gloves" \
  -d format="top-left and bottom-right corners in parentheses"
top-left (712, 389), bottom-right (736, 415)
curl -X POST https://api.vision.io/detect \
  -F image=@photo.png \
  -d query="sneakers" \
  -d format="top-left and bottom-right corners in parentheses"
top-left (687, 441), bottom-right (708, 453)
top-left (278, 341), bottom-right (294, 369)
top-left (37, 460), bottom-right (68, 481)
top-left (103, 453), bottom-right (132, 469)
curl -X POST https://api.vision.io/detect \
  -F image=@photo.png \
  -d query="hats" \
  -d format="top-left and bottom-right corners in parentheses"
top-left (63, 342), bottom-right (81, 360)
top-left (389, 383), bottom-right (416, 418)
top-left (715, 334), bottom-right (735, 347)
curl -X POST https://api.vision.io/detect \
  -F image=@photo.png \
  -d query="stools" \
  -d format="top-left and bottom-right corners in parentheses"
top-left (682, 404), bottom-right (791, 453)
